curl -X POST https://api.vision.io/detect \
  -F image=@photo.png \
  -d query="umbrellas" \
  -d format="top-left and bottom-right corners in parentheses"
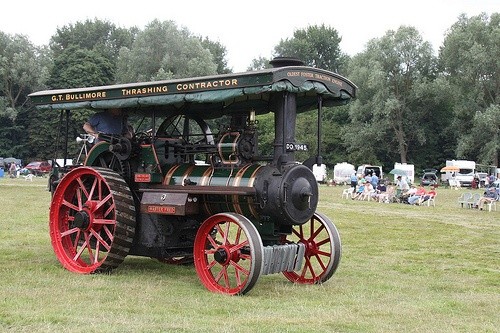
top-left (441, 167), bottom-right (460, 172)
top-left (389, 168), bottom-right (406, 176)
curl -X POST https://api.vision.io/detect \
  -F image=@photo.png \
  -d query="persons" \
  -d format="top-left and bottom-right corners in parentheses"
top-left (351, 170), bottom-right (498, 211)
top-left (7, 162), bottom-right (20, 179)
top-left (83, 109), bottom-right (133, 144)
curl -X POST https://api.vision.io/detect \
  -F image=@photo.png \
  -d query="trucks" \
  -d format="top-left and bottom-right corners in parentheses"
top-left (445, 159), bottom-right (476, 187)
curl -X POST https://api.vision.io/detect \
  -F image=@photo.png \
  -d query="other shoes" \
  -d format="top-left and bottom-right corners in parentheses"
top-left (472, 205), bottom-right (478, 208)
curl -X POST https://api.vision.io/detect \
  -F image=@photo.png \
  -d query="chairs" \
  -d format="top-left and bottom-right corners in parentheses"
top-left (344, 187), bottom-right (436, 206)
top-left (24, 173), bottom-right (34, 182)
top-left (459, 191), bottom-right (496, 211)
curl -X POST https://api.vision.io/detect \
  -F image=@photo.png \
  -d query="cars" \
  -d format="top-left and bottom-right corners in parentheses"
top-left (24, 161), bottom-right (52, 176)
top-left (475, 173), bottom-right (489, 188)
top-left (419, 172), bottom-right (439, 186)
